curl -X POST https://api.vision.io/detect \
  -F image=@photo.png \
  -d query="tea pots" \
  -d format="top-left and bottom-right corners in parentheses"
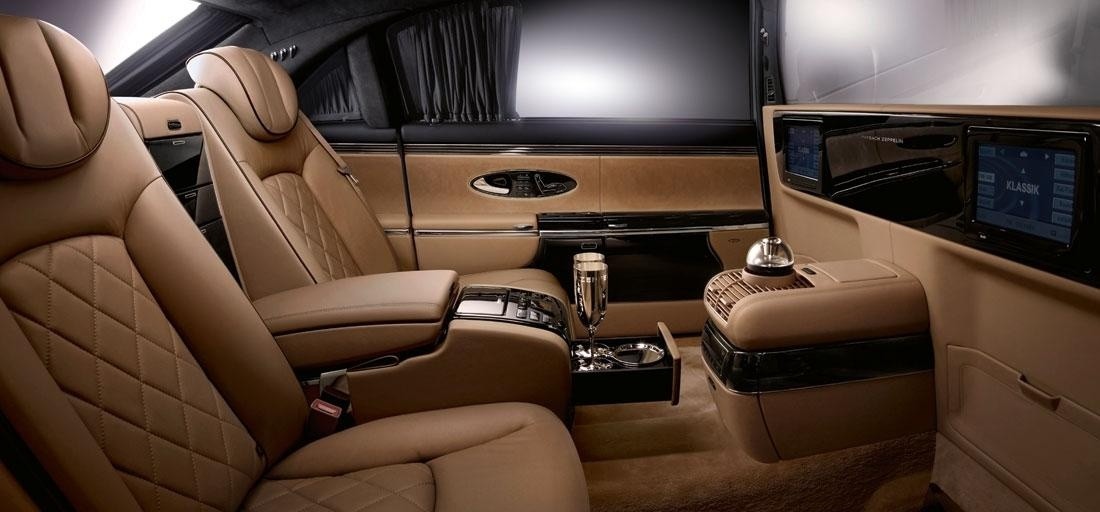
top-left (745, 236), bottom-right (795, 275)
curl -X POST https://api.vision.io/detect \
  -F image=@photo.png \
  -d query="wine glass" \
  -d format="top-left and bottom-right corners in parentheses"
top-left (567, 252), bottom-right (613, 372)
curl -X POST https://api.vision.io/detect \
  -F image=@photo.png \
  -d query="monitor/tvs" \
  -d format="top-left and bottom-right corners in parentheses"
top-left (778, 118), bottom-right (824, 190)
top-left (968, 125), bottom-right (1090, 253)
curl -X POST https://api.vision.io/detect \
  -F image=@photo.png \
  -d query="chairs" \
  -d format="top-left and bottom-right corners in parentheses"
top-left (0, 14), bottom-right (593, 510)
